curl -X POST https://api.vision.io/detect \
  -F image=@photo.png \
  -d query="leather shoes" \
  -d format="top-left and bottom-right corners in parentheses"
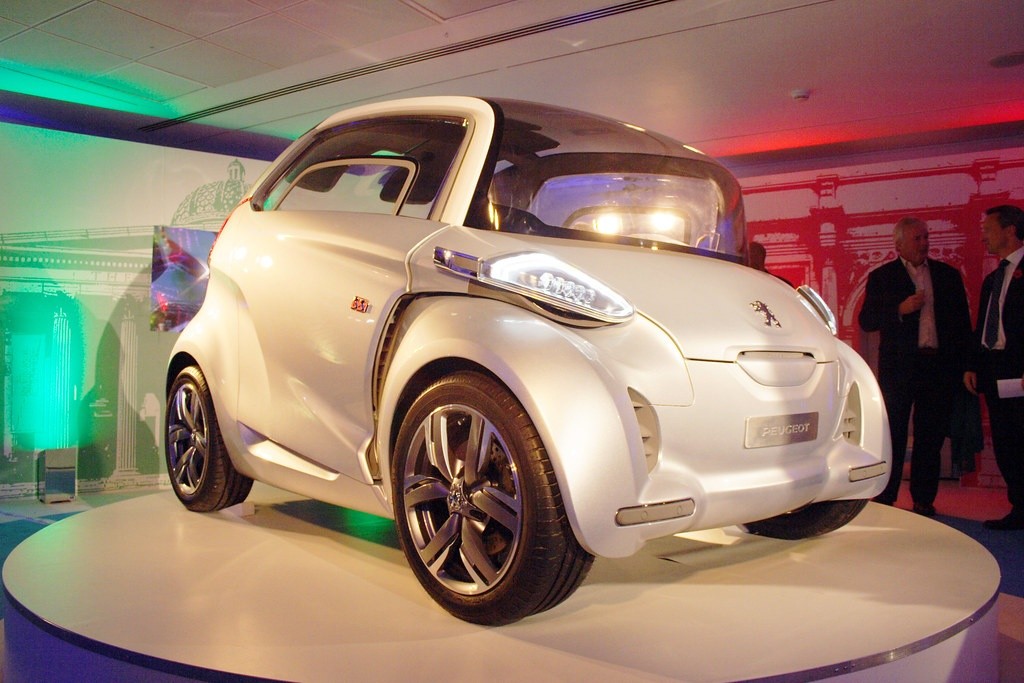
top-left (913, 502), bottom-right (935, 515)
top-left (872, 497), bottom-right (892, 506)
top-left (982, 509), bottom-right (1024, 530)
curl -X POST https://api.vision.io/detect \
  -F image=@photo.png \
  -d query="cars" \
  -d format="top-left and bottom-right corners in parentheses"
top-left (165, 92), bottom-right (893, 629)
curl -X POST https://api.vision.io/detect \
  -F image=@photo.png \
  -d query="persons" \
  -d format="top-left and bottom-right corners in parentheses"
top-left (858, 216), bottom-right (974, 516)
top-left (962, 204), bottom-right (1024, 531)
top-left (740, 241), bottom-right (795, 291)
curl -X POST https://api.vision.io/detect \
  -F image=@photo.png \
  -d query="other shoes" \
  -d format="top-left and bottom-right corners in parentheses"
top-left (967, 458), bottom-right (976, 472)
top-left (951, 465), bottom-right (963, 478)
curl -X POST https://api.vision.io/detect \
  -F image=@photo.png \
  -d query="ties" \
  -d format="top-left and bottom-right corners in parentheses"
top-left (984, 258), bottom-right (1012, 350)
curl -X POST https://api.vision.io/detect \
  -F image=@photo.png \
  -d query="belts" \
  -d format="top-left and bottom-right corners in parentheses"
top-left (916, 348), bottom-right (939, 355)
